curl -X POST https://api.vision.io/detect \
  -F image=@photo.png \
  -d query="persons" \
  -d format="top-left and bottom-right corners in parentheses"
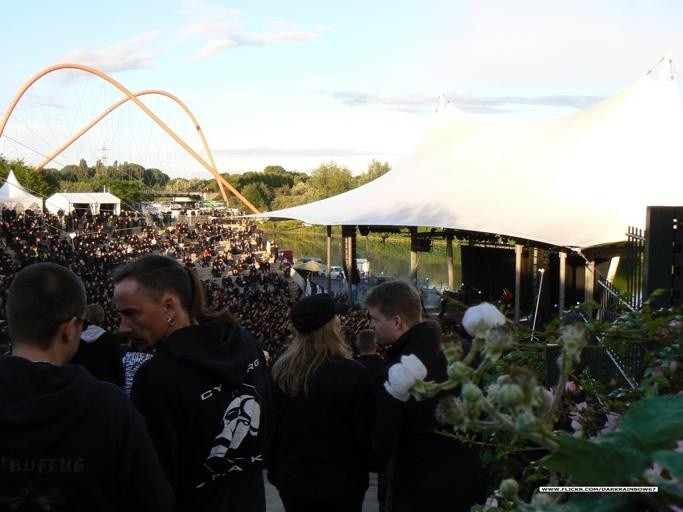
top-left (256, 294), bottom-right (377, 512)
top-left (356, 274), bottom-right (458, 512)
top-left (0, 259), bottom-right (175, 512)
top-left (110, 255), bottom-right (268, 512)
top-left (0, 206), bottom-right (385, 392)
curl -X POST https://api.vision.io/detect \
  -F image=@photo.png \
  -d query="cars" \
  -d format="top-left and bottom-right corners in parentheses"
top-left (297, 257), bottom-right (347, 281)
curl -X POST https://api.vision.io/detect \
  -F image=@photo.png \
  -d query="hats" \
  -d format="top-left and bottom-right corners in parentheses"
top-left (86, 304), bottom-right (105, 323)
top-left (291, 293), bottom-right (346, 332)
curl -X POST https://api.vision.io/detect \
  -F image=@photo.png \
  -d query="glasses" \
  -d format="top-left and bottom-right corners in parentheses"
top-left (78, 318), bottom-right (89, 330)
